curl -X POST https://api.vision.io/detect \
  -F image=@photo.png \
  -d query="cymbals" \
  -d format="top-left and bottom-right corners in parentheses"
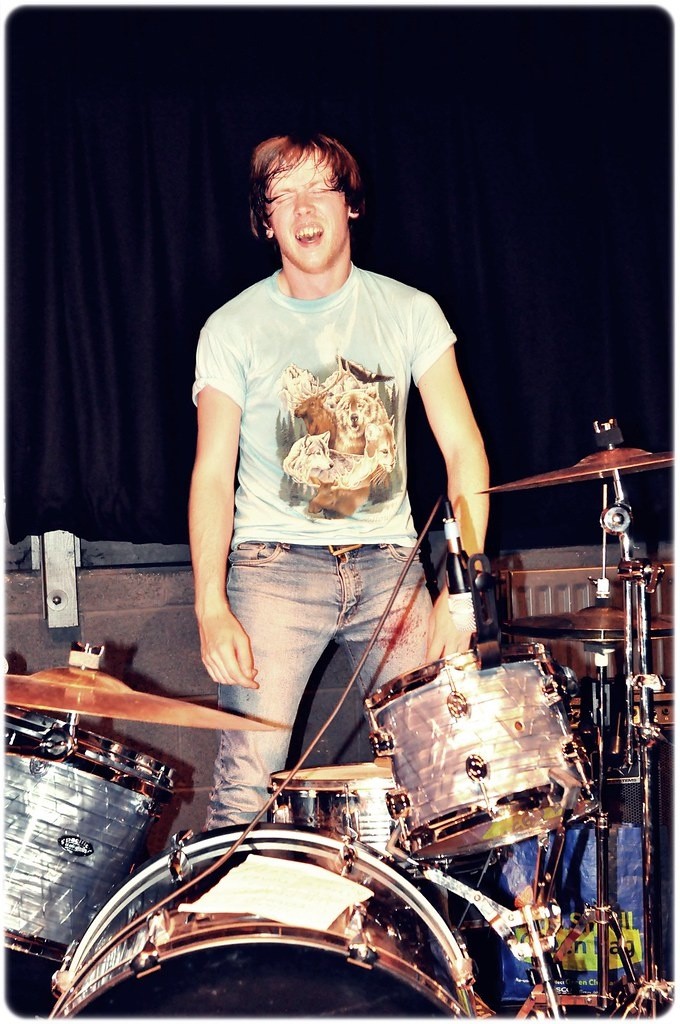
top-left (4, 666), bottom-right (278, 732)
top-left (474, 447), bottom-right (674, 494)
top-left (498, 606), bottom-right (675, 644)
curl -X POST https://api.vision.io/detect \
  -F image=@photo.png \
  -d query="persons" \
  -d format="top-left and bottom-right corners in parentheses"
top-left (184, 131), bottom-right (495, 833)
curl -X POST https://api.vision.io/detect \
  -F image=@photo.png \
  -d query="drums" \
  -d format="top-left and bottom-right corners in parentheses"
top-left (361, 637), bottom-right (599, 863)
top-left (52, 819), bottom-right (477, 1020)
top-left (5, 703), bottom-right (181, 963)
top-left (268, 761), bottom-right (449, 913)
